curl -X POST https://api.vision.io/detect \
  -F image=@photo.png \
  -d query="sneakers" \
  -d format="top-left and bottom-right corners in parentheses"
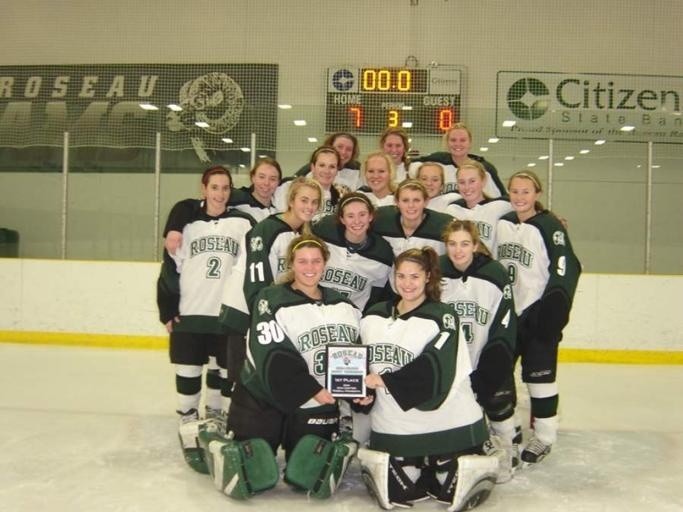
top-left (521, 438), bottom-right (553, 464)
top-left (512, 433), bottom-right (523, 468)
top-left (177, 408), bottom-right (200, 427)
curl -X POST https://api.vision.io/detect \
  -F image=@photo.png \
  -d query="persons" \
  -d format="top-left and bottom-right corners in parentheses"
top-left (206, 235), bottom-right (374, 501)
top-left (271, 123), bottom-right (506, 221)
top-left (359, 245), bottom-right (498, 511)
top-left (162, 157), bottom-right (282, 255)
top-left (369, 179), bottom-right (452, 256)
top-left (493, 171), bottom-right (582, 469)
top-left (312, 193), bottom-right (396, 312)
top-left (216, 177), bottom-right (324, 442)
top-left (438, 221), bottom-right (523, 484)
top-left (156, 166), bottom-right (257, 468)
